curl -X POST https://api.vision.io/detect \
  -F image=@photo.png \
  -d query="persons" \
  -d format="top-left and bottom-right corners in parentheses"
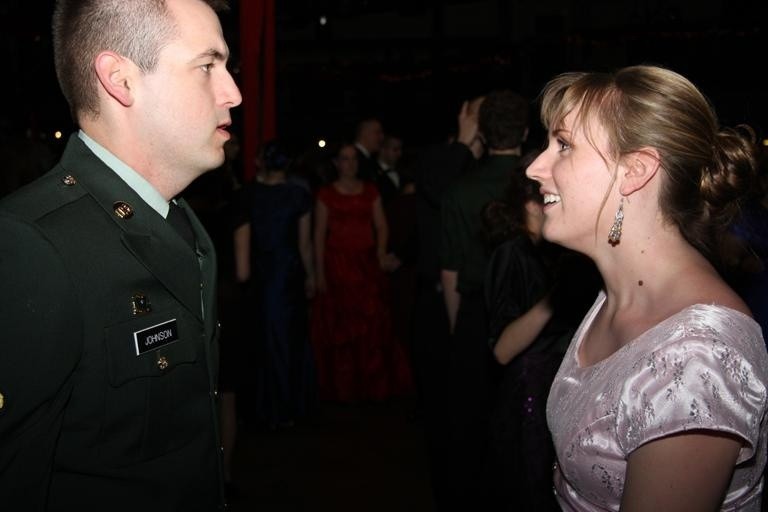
top-left (2, 1), bottom-right (242, 508)
top-left (714, 156), bottom-right (768, 348)
top-left (524, 61), bottom-right (768, 512)
top-left (193, 88), bottom-right (601, 484)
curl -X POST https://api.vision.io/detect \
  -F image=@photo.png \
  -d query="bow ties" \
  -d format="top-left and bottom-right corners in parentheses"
top-left (381, 167), bottom-right (397, 176)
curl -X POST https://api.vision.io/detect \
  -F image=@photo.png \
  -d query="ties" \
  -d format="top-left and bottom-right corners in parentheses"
top-left (163, 203), bottom-right (201, 256)
